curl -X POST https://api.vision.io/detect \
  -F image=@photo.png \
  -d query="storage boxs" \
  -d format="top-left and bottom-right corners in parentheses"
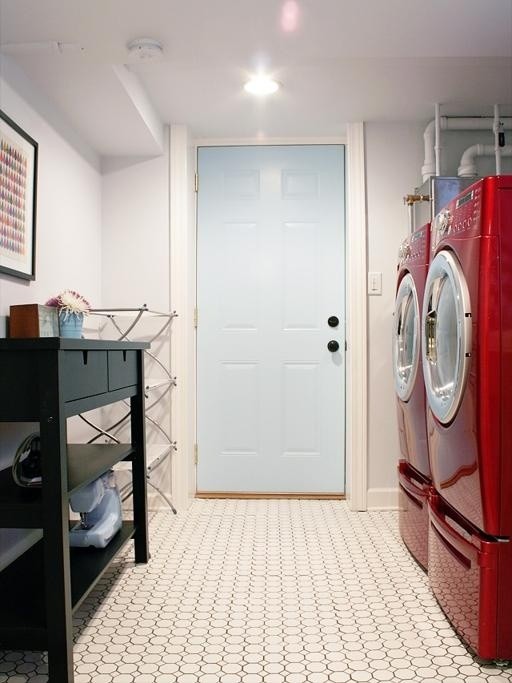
top-left (8, 301), bottom-right (61, 339)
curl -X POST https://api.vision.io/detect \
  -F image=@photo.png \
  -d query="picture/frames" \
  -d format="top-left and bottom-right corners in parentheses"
top-left (0, 106), bottom-right (41, 283)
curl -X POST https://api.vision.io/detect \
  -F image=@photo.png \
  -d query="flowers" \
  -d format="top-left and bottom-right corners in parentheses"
top-left (47, 289), bottom-right (94, 318)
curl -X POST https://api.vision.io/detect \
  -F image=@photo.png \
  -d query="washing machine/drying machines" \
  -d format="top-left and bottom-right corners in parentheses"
top-left (392, 225), bottom-right (429, 572)
top-left (422, 176), bottom-right (511, 662)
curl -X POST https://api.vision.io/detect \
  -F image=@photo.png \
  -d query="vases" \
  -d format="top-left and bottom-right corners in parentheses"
top-left (61, 309), bottom-right (86, 341)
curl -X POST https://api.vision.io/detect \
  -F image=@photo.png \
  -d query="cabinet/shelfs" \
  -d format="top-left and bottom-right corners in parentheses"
top-left (1, 337), bottom-right (155, 682)
top-left (75, 303), bottom-right (181, 519)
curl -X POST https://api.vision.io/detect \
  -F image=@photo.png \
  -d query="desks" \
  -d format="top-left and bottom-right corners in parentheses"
top-left (2, 527), bottom-right (44, 576)
